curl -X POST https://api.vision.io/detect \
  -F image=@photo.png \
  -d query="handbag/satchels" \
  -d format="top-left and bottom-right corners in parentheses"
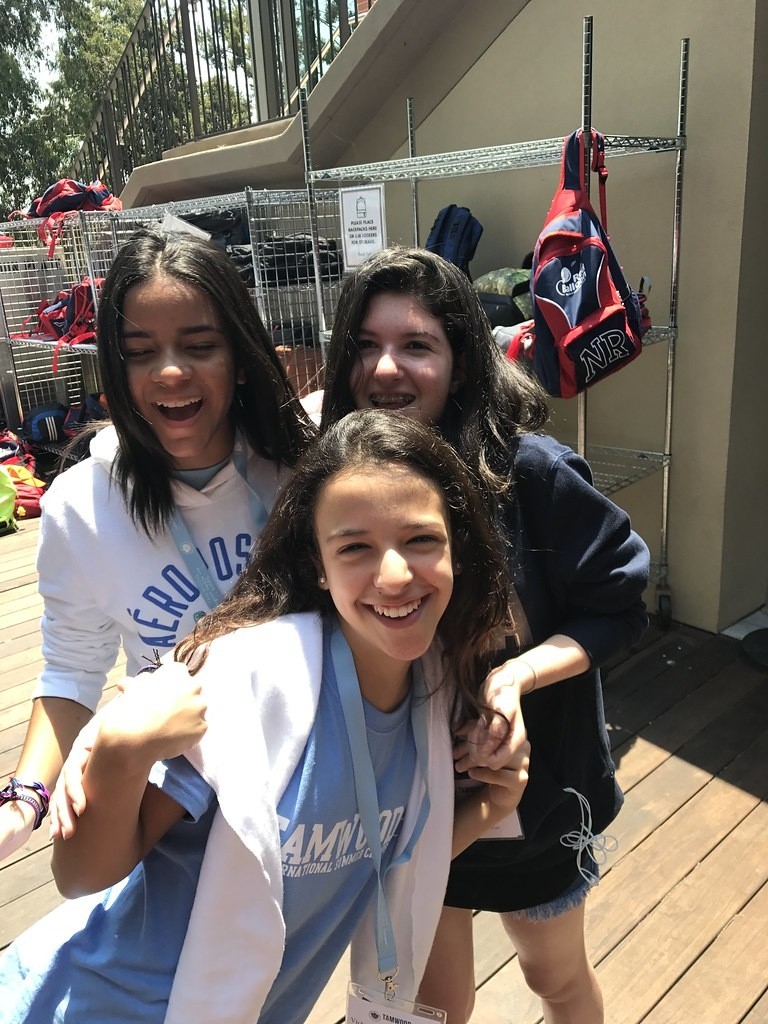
top-left (180, 209), bottom-right (343, 287)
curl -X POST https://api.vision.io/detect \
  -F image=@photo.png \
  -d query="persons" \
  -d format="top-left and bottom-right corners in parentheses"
top-left (49, 244), bottom-right (651, 1024)
top-left (0, 409), bottom-right (531, 1024)
top-left (0, 231), bottom-right (320, 863)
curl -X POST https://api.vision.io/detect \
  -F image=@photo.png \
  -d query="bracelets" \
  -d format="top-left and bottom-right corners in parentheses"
top-left (515, 658), bottom-right (536, 694)
top-left (0, 776), bottom-right (51, 830)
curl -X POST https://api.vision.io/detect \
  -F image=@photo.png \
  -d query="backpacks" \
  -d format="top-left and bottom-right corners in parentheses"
top-left (423, 126), bottom-right (651, 399)
top-left (0, 390), bottom-right (114, 537)
top-left (7, 178), bottom-right (123, 257)
top-left (11, 276), bottom-right (106, 344)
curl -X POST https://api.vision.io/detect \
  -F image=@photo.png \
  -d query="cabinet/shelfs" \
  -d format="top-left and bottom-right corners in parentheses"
top-left (0, 183), bottom-right (339, 470)
top-left (296, 11), bottom-right (690, 633)
